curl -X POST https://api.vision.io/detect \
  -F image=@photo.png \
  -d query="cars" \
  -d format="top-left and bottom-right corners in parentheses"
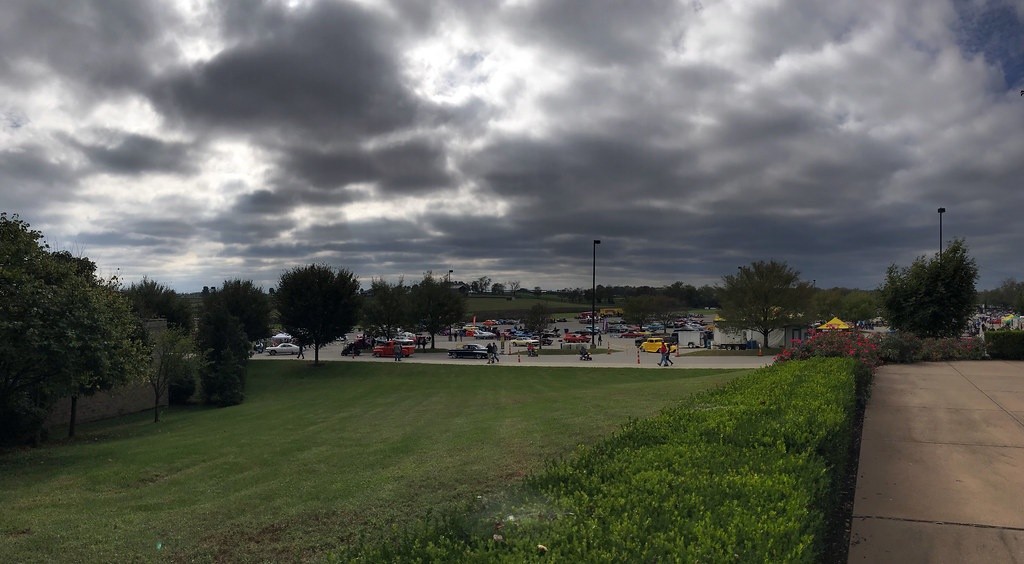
top-left (441, 318), bottom-right (560, 343)
top-left (563, 333), bottom-right (590, 344)
top-left (640, 337), bottom-right (677, 354)
top-left (448, 343), bottom-right (488, 360)
top-left (584, 326), bottom-right (599, 335)
top-left (512, 337), bottom-right (541, 347)
top-left (265, 343), bottom-right (306, 356)
top-left (608, 320), bottom-right (665, 338)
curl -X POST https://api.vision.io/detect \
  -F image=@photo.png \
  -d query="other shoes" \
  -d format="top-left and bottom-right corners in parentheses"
top-left (487, 362), bottom-right (490, 364)
top-left (491, 361), bottom-right (493, 363)
top-left (395, 360), bottom-right (397, 361)
top-left (664, 364), bottom-right (668, 366)
top-left (398, 359), bottom-right (402, 361)
top-left (657, 363), bottom-right (661, 366)
top-left (497, 360), bottom-right (499, 362)
top-left (671, 363), bottom-right (673, 365)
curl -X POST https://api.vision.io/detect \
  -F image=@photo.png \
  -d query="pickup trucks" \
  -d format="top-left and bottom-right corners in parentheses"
top-left (374, 340), bottom-right (415, 358)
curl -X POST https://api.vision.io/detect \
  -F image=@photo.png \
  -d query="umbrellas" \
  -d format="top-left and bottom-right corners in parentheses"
top-left (481, 321), bottom-right (496, 329)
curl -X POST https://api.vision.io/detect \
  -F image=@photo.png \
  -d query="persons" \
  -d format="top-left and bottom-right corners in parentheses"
top-left (438, 330), bottom-right (463, 342)
top-left (962, 312), bottom-right (1022, 339)
top-left (415, 334), bottom-right (432, 350)
top-left (297, 334), bottom-right (376, 359)
top-left (857, 317), bottom-right (888, 333)
top-left (586, 310), bottom-right (621, 324)
top-left (485, 320), bottom-right (602, 364)
top-left (254, 340), bottom-right (283, 353)
top-left (657, 341), bottom-right (673, 366)
top-left (700, 333), bottom-right (708, 348)
top-left (394, 341), bottom-right (403, 362)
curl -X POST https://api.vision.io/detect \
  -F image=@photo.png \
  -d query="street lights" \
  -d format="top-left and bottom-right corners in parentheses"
top-left (449, 269), bottom-right (454, 283)
top-left (937, 208), bottom-right (946, 265)
top-left (590, 239), bottom-right (602, 349)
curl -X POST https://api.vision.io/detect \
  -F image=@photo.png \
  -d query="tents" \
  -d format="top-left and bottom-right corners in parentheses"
top-left (272, 333), bottom-right (290, 339)
top-left (815, 316), bottom-right (851, 330)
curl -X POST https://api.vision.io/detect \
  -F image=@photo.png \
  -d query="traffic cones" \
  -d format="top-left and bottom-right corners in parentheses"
top-left (758, 344), bottom-right (763, 356)
